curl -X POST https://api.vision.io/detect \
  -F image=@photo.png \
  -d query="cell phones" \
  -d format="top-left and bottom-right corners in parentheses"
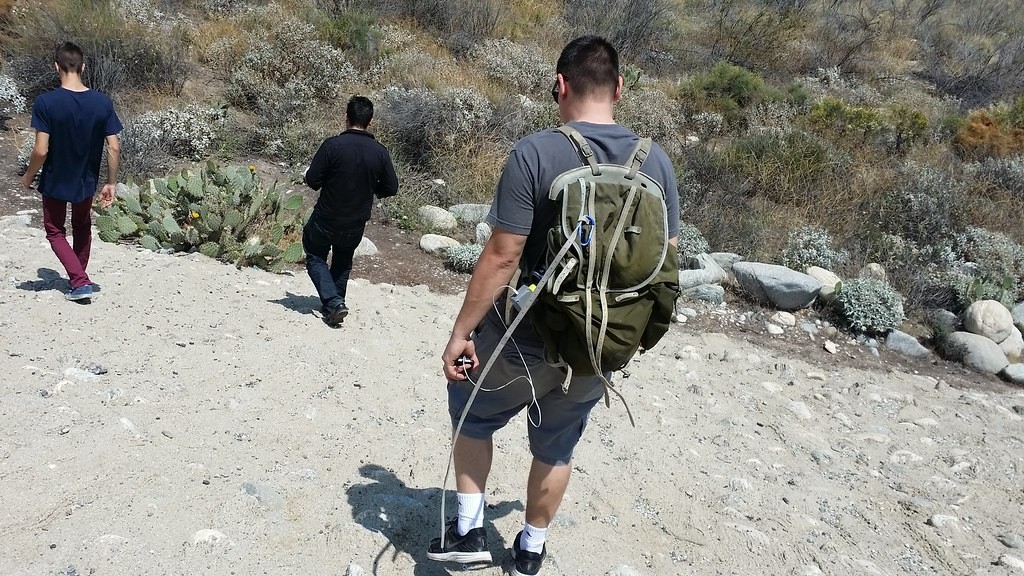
top-left (456, 359), bottom-right (474, 382)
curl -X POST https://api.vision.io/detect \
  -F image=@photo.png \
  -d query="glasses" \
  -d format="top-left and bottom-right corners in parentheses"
top-left (551, 75), bottom-right (569, 103)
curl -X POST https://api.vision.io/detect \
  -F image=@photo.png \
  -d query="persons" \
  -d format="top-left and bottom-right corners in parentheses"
top-left (302, 96), bottom-right (399, 326)
top-left (426, 34), bottom-right (682, 576)
top-left (21, 41), bottom-right (124, 301)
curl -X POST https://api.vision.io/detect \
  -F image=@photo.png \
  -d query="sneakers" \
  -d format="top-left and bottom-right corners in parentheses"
top-left (511, 530), bottom-right (547, 576)
top-left (426, 518), bottom-right (492, 564)
top-left (322, 303), bottom-right (349, 326)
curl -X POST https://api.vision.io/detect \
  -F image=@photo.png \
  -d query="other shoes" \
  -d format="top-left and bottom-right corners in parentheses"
top-left (65, 280), bottom-right (93, 300)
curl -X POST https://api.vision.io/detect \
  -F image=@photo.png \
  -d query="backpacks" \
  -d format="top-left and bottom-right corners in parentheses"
top-left (506, 126), bottom-right (681, 427)
